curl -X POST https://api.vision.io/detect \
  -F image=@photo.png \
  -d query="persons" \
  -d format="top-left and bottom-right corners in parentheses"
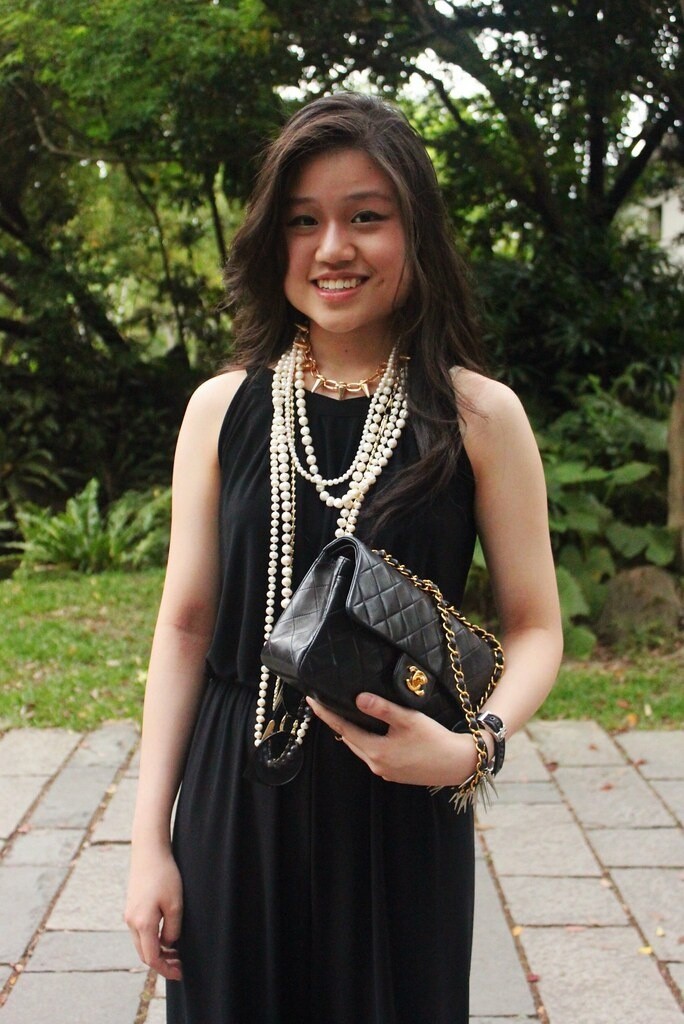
top-left (124, 94), bottom-right (564, 1024)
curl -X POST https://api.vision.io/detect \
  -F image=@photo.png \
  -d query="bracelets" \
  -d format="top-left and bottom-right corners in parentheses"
top-left (426, 712), bottom-right (508, 813)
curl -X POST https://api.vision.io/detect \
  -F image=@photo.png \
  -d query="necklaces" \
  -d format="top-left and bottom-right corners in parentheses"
top-left (253, 322), bottom-right (408, 770)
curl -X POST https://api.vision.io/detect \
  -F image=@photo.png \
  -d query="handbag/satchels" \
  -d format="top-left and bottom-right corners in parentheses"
top-left (260, 536), bottom-right (504, 792)
